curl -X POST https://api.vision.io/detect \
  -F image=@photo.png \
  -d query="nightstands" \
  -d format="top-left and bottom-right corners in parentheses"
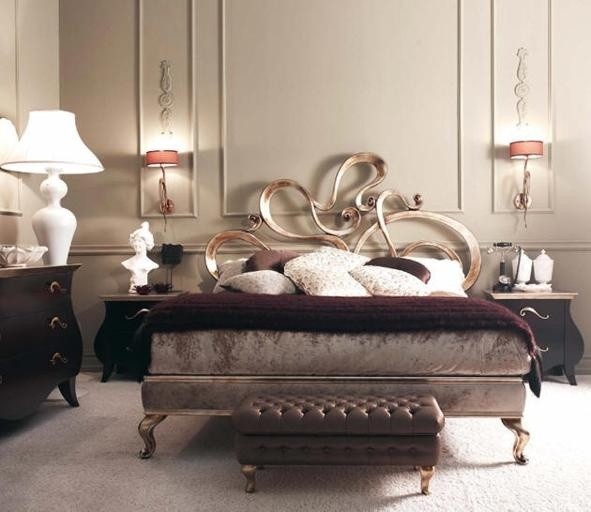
top-left (482, 288), bottom-right (584, 386)
top-left (94, 290), bottom-right (193, 384)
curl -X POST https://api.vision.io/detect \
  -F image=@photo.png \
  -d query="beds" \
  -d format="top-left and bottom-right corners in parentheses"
top-left (138, 152), bottom-right (534, 465)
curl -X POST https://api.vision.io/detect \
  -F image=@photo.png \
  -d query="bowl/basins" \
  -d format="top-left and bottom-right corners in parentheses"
top-left (0, 244), bottom-right (49, 267)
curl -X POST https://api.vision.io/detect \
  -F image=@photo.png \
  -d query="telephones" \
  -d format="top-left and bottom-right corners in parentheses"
top-left (487, 241), bottom-right (520, 293)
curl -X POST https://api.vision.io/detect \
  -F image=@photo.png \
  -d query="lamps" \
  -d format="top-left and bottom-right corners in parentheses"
top-left (509, 140), bottom-right (544, 210)
top-left (145, 149), bottom-right (179, 214)
top-left (1, 109), bottom-right (105, 267)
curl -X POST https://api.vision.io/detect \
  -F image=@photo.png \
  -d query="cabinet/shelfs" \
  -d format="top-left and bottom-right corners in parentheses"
top-left (0, 264), bottom-right (82, 421)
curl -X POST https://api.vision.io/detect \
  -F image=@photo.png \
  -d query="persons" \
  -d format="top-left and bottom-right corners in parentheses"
top-left (119, 220), bottom-right (161, 294)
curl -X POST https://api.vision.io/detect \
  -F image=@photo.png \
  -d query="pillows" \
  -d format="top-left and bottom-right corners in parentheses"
top-left (212, 250), bottom-right (469, 297)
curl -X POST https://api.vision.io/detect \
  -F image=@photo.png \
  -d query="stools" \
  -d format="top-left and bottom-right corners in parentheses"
top-left (231, 392), bottom-right (445, 496)
